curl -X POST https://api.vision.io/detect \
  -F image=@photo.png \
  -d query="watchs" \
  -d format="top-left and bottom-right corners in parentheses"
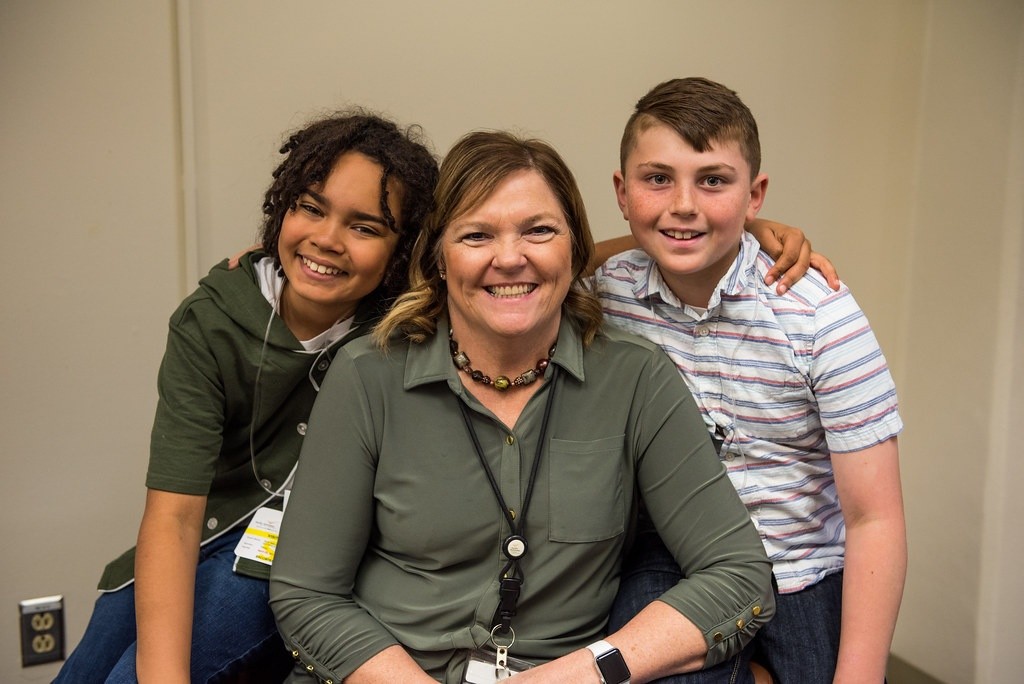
top-left (586, 640), bottom-right (631, 684)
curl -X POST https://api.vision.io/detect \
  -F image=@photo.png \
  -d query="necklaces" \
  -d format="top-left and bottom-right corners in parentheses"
top-left (449, 324), bottom-right (557, 392)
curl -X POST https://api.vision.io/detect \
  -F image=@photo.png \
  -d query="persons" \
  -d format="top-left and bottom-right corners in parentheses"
top-left (49, 116), bottom-right (841, 684)
top-left (577, 77), bottom-right (908, 684)
top-left (269, 132), bottom-right (778, 684)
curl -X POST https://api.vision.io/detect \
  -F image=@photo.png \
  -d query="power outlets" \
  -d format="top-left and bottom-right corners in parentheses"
top-left (18, 595), bottom-right (66, 668)
